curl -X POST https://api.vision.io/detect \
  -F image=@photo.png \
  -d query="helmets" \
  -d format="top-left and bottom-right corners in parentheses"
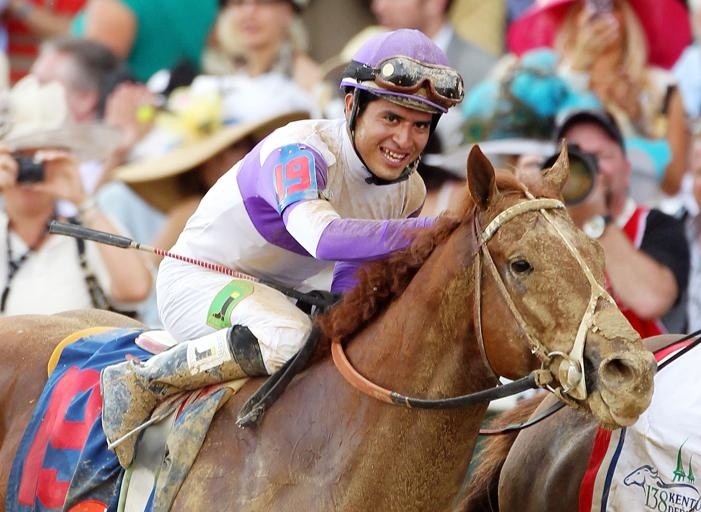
top-left (339, 27), bottom-right (464, 113)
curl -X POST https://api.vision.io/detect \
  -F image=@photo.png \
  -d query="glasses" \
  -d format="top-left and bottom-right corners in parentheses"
top-left (346, 55), bottom-right (465, 107)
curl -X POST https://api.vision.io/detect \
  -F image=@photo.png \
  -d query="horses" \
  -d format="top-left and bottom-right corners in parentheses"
top-left (0, 135), bottom-right (658, 511)
top-left (456, 333), bottom-right (700, 511)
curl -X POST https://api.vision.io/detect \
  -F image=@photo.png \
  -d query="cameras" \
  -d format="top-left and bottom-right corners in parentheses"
top-left (11, 155), bottom-right (46, 184)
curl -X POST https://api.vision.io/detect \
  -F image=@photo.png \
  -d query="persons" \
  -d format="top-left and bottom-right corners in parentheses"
top-left (93, 26), bottom-right (471, 474)
top-left (1, 3), bottom-right (701, 412)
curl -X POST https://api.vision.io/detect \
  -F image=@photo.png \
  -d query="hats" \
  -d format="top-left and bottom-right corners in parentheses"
top-left (116, 91), bottom-right (314, 214)
top-left (551, 106), bottom-right (622, 155)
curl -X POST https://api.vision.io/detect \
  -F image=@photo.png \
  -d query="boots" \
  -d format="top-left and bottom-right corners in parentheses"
top-left (100, 324), bottom-right (249, 471)
